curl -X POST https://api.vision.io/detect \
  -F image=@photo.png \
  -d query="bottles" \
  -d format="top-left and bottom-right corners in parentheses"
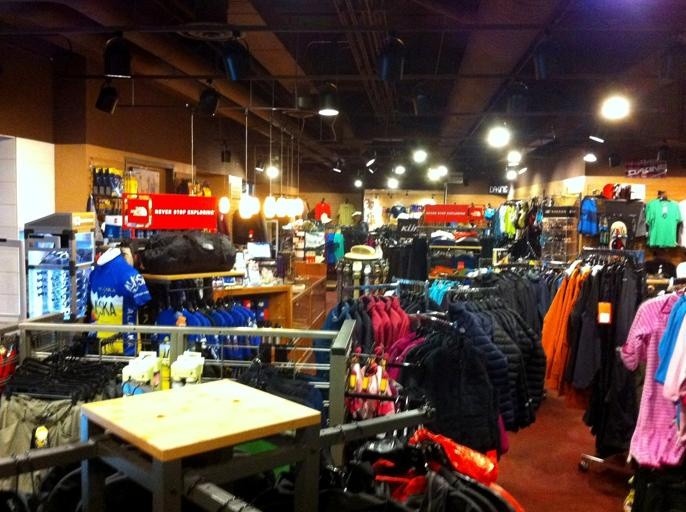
top-left (123, 166), bottom-right (139, 199)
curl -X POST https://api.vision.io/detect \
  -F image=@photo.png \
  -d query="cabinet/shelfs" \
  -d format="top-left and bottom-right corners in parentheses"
top-left (23, 212), bottom-right (97, 320)
top-left (289, 263), bottom-right (328, 375)
top-left (278, 228), bottom-right (326, 259)
top-left (540, 206), bottom-right (578, 261)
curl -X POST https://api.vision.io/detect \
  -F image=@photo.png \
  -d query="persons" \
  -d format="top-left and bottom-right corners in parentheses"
top-left (89, 246), bottom-right (151, 360)
top-left (349, 211), bottom-right (370, 233)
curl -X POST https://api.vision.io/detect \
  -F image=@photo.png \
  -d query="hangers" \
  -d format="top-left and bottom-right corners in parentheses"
top-left (159, 284), bottom-right (266, 311)
top-left (3, 342), bottom-right (273, 413)
top-left (659, 191), bottom-right (671, 202)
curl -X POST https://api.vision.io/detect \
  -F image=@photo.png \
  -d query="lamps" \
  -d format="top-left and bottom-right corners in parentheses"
top-left (317, 89), bottom-right (339, 116)
top-left (379, 32), bottom-right (411, 80)
top-left (95, 79), bottom-right (120, 115)
top-left (198, 78), bottom-right (220, 120)
top-left (101, 31), bottom-right (132, 78)
top-left (218, 108), bottom-right (304, 219)
top-left (534, 28), bottom-right (565, 81)
top-left (221, 29), bottom-right (251, 81)
